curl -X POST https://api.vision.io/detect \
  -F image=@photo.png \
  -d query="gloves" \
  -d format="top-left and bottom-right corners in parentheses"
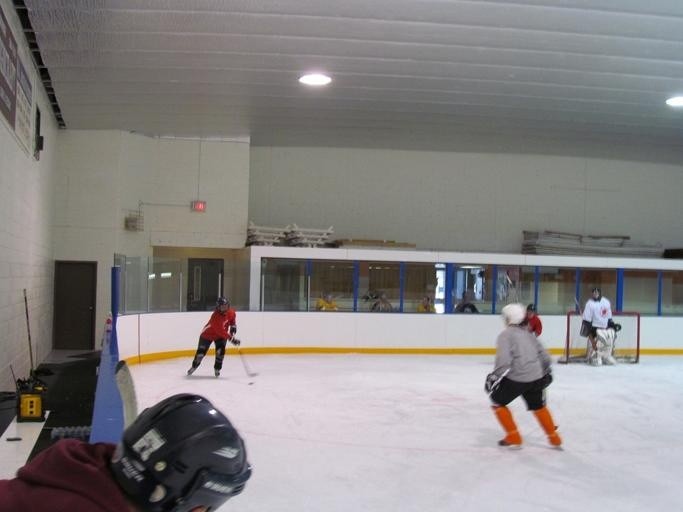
top-left (230, 325), bottom-right (236, 335)
top-left (229, 337), bottom-right (240, 345)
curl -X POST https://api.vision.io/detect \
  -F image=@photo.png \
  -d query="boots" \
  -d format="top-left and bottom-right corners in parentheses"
top-left (495, 407), bottom-right (522, 445)
top-left (534, 406), bottom-right (561, 444)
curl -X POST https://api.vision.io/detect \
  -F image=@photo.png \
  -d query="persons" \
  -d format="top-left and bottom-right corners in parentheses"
top-left (483, 301), bottom-right (563, 449)
top-left (187, 296), bottom-right (241, 378)
top-left (315, 290), bottom-right (339, 311)
top-left (518, 303), bottom-right (542, 339)
top-left (0, 392), bottom-right (251, 512)
top-left (369, 290), bottom-right (394, 312)
top-left (452, 290), bottom-right (482, 314)
top-left (415, 295), bottom-right (436, 313)
top-left (580, 286), bottom-right (621, 367)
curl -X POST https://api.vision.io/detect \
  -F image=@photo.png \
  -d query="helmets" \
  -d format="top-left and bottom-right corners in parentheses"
top-left (502, 304), bottom-right (526, 325)
top-left (216, 298), bottom-right (227, 307)
top-left (110, 394), bottom-right (251, 512)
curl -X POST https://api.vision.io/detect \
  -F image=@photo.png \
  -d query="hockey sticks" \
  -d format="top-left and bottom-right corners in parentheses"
top-left (571, 295), bottom-right (610, 353)
top-left (232, 331), bottom-right (261, 377)
